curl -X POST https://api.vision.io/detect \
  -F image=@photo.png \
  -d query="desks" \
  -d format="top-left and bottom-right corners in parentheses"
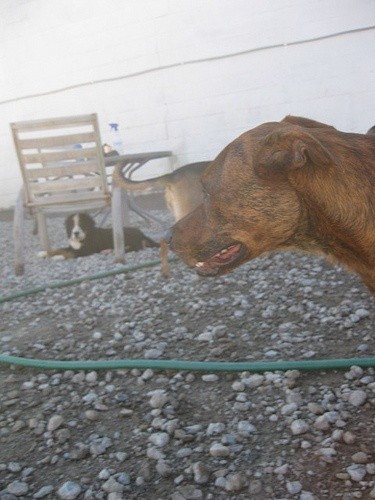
top-left (12, 151), bottom-right (174, 276)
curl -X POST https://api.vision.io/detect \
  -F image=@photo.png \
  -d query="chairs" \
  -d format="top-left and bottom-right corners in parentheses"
top-left (9, 113), bottom-right (112, 257)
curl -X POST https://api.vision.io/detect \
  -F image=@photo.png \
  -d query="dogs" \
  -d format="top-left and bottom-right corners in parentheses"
top-left (38, 211), bottom-right (161, 261)
top-left (113, 160), bottom-right (217, 278)
top-left (163, 115), bottom-right (375, 302)
